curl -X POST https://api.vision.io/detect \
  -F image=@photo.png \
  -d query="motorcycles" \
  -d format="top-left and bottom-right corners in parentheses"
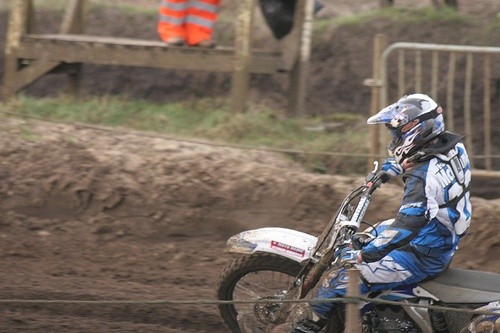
top-left (214, 161), bottom-right (500, 333)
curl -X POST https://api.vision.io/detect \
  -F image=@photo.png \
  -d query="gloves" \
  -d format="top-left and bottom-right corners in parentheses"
top-left (381, 157), bottom-right (404, 179)
top-left (338, 247), bottom-right (361, 269)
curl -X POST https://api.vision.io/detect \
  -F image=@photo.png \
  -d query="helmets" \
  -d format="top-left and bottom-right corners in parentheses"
top-left (367, 93), bottom-right (445, 164)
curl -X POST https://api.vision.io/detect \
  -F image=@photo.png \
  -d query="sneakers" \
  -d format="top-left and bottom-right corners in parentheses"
top-left (290, 318), bottom-right (330, 333)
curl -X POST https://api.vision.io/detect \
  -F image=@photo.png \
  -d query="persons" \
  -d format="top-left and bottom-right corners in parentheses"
top-left (158, 0), bottom-right (221, 48)
top-left (291, 93), bottom-right (473, 333)
